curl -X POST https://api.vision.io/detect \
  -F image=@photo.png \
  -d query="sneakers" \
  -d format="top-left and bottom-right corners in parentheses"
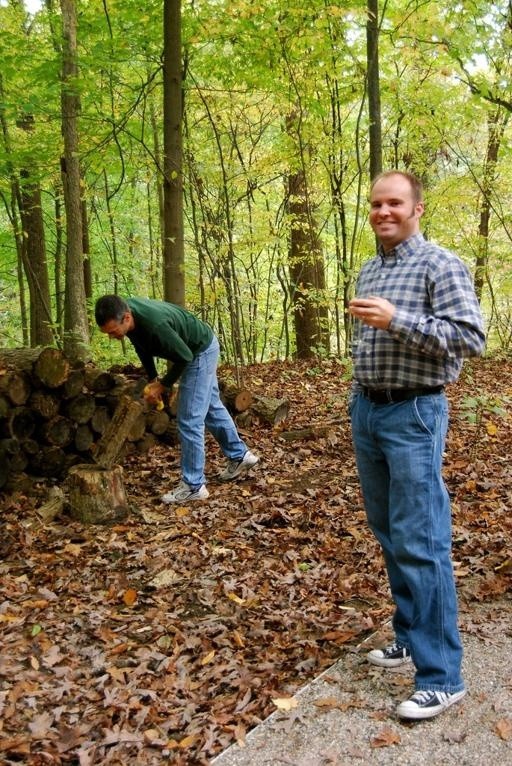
top-left (160, 481), bottom-right (209, 504)
top-left (367, 644), bottom-right (411, 667)
top-left (218, 451), bottom-right (258, 482)
top-left (397, 690), bottom-right (466, 719)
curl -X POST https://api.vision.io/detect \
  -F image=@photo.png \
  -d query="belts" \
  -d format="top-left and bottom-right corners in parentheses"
top-left (350, 385), bottom-right (443, 404)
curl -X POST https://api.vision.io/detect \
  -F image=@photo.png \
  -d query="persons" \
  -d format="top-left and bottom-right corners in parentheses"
top-left (346, 171), bottom-right (486, 719)
top-left (95, 294), bottom-right (259, 503)
